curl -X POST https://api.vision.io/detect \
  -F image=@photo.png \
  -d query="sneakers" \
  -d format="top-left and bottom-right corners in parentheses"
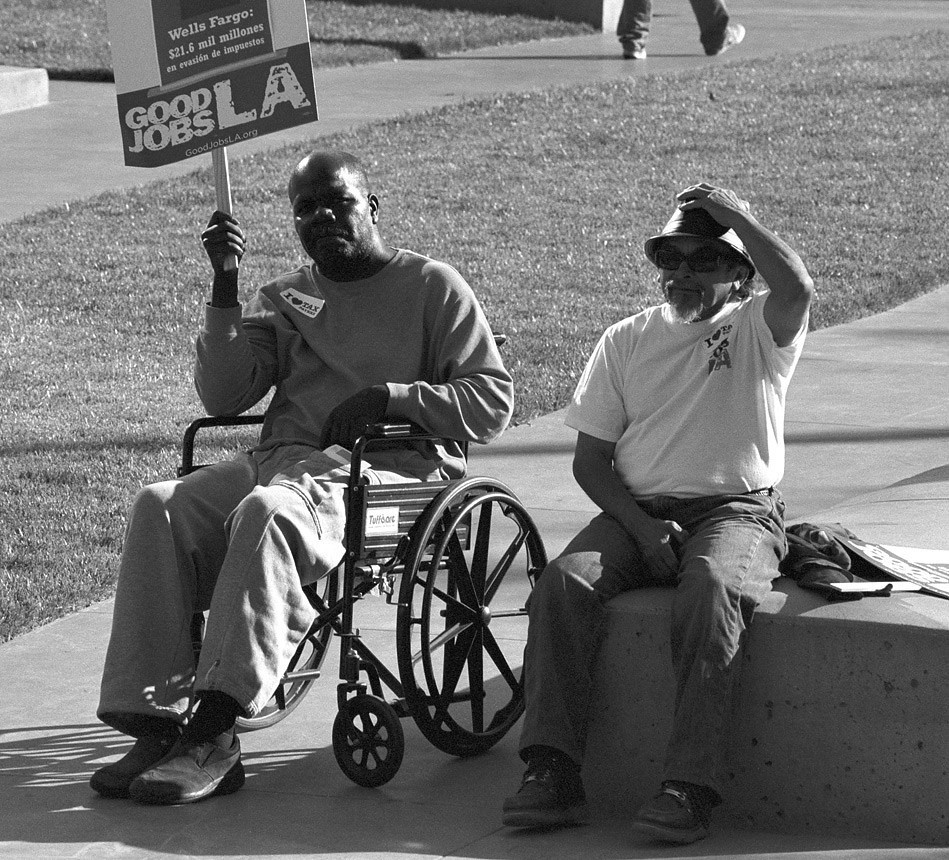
top-left (501, 751), bottom-right (593, 826)
top-left (129, 730), bottom-right (247, 804)
top-left (632, 780), bottom-right (713, 843)
top-left (89, 735), bottom-right (176, 798)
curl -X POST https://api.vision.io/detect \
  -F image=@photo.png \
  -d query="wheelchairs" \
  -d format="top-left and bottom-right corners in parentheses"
top-left (175, 415), bottom-right (553, 791)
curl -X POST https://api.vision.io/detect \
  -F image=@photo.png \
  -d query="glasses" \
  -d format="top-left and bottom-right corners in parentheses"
top-left (653, 243), bottom-right (737, 273)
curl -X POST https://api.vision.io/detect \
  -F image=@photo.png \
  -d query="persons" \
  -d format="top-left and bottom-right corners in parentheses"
top-left (88, 150), bottom-right (516, 807)
top-left (614, 0), bottom-right (750, 61)
top-left (494, 182), bottom-right (821, 849)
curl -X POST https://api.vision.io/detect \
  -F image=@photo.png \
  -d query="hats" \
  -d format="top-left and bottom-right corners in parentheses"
top-left (644, 200), bottom-right (756, 280)
